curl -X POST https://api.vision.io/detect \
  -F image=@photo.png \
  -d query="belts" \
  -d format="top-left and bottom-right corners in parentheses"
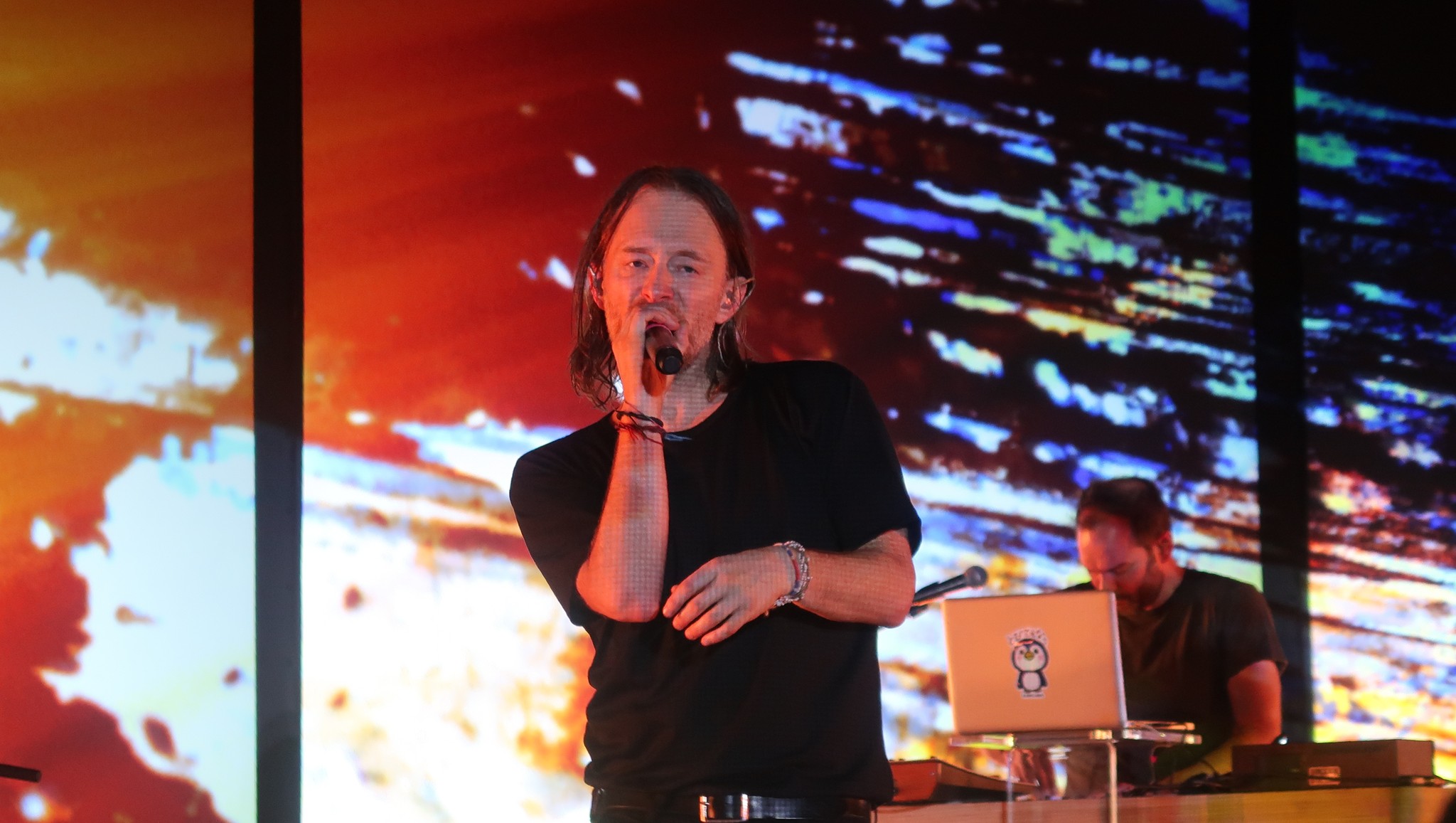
top-left (593, 790), bottom-right (872, 822)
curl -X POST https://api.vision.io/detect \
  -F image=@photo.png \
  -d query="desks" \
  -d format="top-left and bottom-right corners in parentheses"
top-left (947, 728), bottom-right (1209, 823)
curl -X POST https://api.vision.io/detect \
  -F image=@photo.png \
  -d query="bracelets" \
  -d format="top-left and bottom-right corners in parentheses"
top-left (611, 410), bottom-right (667, 437)
top-left (615, 408), bottom-right (665, 427)
top-left (765, 539), bottom-right (812, 615)
top-left (1200, 759), bottom-right (1222, 778)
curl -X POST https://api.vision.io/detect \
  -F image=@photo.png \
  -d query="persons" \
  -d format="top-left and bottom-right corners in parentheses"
top-left (509, 162), bottom-right (924, 823)
top-left (1049, 476), bottom-right (1291, 802)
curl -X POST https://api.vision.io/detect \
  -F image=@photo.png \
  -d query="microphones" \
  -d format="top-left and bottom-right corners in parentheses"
top-left (909, 566), bottom-right (988, 617)
top-left (642, 319), bottom-right (685, 378)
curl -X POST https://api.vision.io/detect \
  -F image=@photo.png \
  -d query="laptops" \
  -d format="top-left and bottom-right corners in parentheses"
top-left (941, 585), bottom-right (1196, 738)
